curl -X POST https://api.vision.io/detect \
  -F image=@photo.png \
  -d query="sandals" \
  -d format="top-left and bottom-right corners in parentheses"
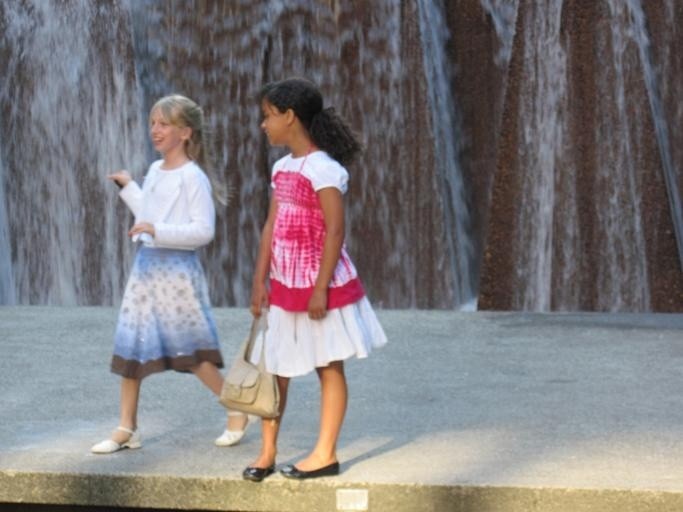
top-left (88, 426), bottom-right (141, 455)
top-left (213, 403), bottom-right (258, 447)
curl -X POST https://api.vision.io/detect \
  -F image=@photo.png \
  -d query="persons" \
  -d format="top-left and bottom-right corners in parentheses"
top-left (90, 94), bottom-right (258, 454)
top-left (242, 78), bottom-right (390, 483)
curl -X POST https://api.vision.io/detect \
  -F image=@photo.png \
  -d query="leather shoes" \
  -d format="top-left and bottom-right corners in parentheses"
top-left (242, 462), bottom-right (277, 483)
top-left (279, 463), bottom-right (340, 480)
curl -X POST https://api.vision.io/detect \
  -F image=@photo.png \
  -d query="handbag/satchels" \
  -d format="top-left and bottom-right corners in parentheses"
top-left (218, 306), bottom-right (280, 420)
